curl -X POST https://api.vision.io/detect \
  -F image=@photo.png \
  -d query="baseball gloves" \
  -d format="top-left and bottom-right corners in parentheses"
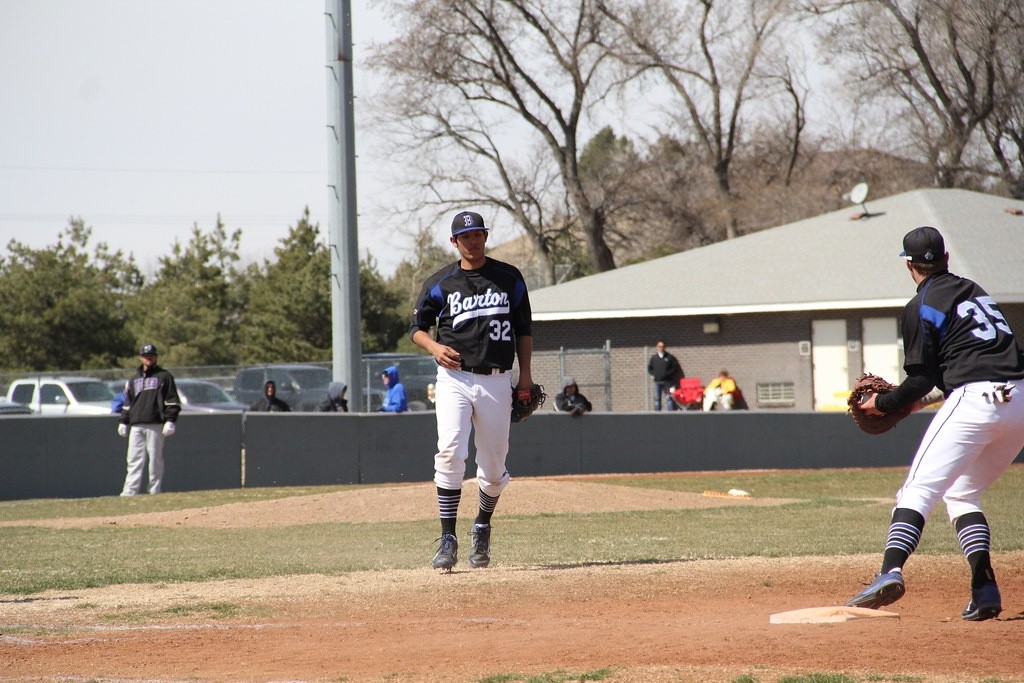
top-left (511, 384), bottom-right (544, 425)
top-left (848, 375), bottom-right (910, 435)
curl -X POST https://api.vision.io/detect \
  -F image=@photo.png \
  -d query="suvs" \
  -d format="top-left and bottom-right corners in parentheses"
top-left (232, 364), bottom-right (386, 413)
top-left (0, 376), bottom-right (130, 419)
top-left (103, 380), bottom-right (251, 413)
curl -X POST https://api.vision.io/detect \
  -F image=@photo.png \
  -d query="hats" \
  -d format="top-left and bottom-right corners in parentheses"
top-left (451, 211), bottom-right (489, 235)
top-left (141, 345), bottom-right (158, 355)
top-left (899, 226), bottom-right (945, 259)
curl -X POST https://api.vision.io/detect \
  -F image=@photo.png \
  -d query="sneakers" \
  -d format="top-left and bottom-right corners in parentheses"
top-left (845, 571), bottom-right (905, 610)
top-left (432, 534), bottom-right (459, 570)
top-left (470, 523), bottom-right (492, 568)
top-left (961, 586), bottom-right (1002, 620)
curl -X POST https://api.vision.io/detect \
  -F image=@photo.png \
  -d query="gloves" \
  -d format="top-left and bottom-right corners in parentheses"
top-left (118, 424), bottom-right (128, 437)
top-left (162, 421), bottom-right (176, 437)
top-left (983, 384), bottom-right (1016, 403)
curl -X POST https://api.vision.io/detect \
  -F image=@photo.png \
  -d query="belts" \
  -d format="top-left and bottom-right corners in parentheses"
top-left (459, 367), bottom-right (505, 375)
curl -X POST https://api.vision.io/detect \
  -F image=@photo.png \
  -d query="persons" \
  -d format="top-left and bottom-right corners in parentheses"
top-left (249, 379), bottom-right (291, 412)
top-left (647, 341), bottom-right (678, 411)
top-left (375, 366), bottom-right (407, 413)
top-left (407, 210), bottom-right (542, 573)
top-left (844, 226), bottom-right (1024, 621)
top-left (117, 345), bottom-right (182, 496)
top-left (110, 379), bottom-right (129, 414)
top-left (313, 382), bottom-right (348, 412)
top-left (702, 369), bottom-right (737, 413)
top-left (553, 377), bottom-right (591, 418)
